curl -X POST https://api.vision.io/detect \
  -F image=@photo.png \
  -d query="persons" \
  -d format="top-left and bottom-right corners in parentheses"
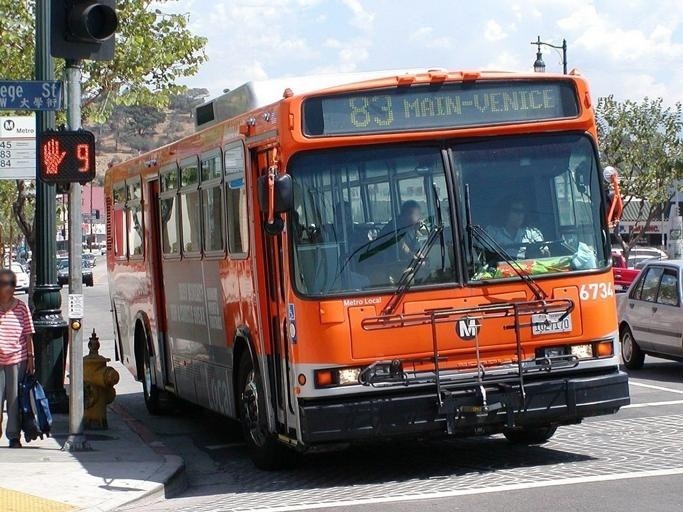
top-left (479, 196), bottom-right (551, 261)
top-left (380, 201), bottom-right (426, 286)
top-left (0, 270), bottom-right (36, 447)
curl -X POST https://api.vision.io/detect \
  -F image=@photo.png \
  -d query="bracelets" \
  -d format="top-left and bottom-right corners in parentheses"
top-left (28, 356), bottom-right (35, 360)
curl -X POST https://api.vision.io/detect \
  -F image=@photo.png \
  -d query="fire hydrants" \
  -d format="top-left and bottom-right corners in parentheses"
top-left (83, 329), bottom-right (120, 432)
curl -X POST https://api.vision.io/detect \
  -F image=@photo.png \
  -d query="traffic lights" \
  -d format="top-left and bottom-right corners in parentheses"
top-left (38, 130), bottom-right (96, 184)
top-left (51, 0), bottom-right (118, 62)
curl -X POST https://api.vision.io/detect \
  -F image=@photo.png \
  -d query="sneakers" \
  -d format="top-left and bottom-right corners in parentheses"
top-left (10, 438), bottom-right (21, 448)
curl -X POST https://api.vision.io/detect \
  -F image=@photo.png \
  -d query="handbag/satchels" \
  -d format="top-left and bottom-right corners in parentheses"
top-left (20, 374), bottom-right (52, 441)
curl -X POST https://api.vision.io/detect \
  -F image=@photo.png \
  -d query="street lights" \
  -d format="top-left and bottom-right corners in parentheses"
top-left (531, 37), bottom-right (568, 75)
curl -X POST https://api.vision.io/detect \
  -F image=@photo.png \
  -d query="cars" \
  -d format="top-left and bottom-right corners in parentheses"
top-left (611, 247), bottom-right (683, 371)
top-left (3, 242), bottom-right (106, 292)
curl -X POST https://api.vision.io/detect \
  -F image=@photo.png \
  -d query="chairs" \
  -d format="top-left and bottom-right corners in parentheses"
top-left (351, 224), bottom-right (384, 272)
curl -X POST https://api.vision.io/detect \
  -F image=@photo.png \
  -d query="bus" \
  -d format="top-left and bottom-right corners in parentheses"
top-left (103, 66), bottom-right (630, 479)
